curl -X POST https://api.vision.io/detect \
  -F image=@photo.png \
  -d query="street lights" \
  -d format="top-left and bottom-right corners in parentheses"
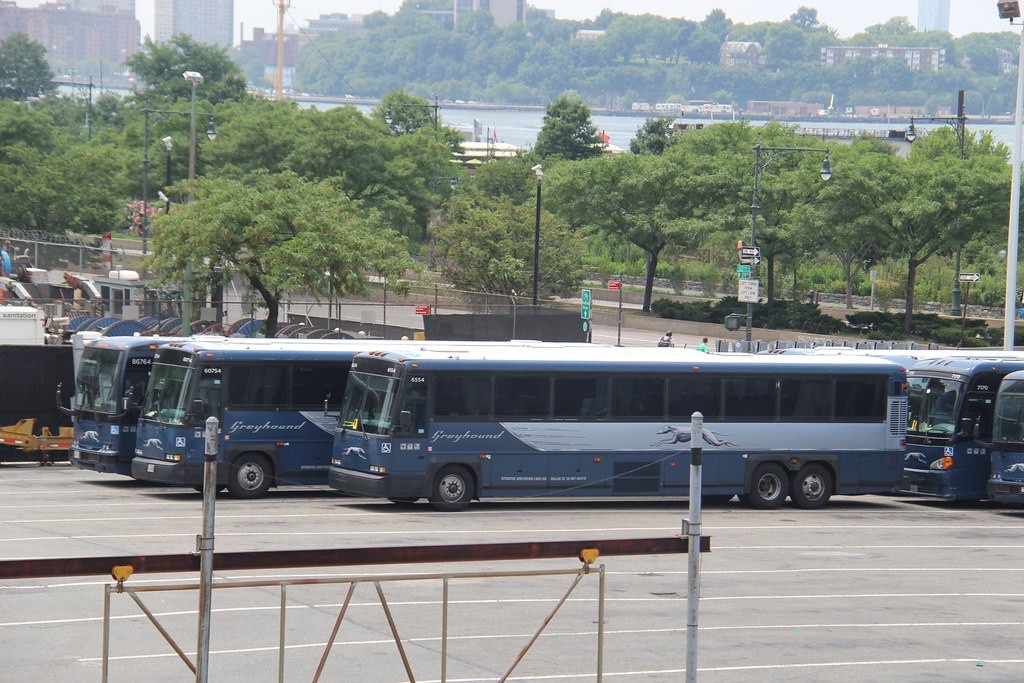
top-left (746, 143), bottom-right (832, 350)
top-left (161, 135), bottom-right (174, 215)
top-left (182, 70), bottom-right (204, 202)
top-left (904, 115), bottom-right (1023, 351)
top-left (530, 163), bottom-right (544, 305)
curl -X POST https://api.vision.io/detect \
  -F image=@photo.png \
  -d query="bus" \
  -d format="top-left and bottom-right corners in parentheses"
top-left (55, 333), bottom-right (542, 485)
top-left (900, 357), bottom-right (1024, 505)
top-left (323, 347), bottom-right (911, 513)
top-left (130, 338), bottom-right (630, 500)
top-left (971, 369), bottom-right (1024, 509)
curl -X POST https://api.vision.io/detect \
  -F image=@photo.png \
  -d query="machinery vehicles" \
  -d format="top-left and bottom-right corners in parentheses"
top-left (0, 251), bottom-right (105, 466)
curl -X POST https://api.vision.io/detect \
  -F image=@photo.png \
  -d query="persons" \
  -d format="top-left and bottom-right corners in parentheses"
top-left (658, 331), bottom-right (674, 347)
top-left (697, 338), bottom-right (709, 351)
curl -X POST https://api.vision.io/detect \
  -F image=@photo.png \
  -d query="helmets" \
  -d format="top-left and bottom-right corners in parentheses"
top-left (666, 331), bottom-right (672, 336)
